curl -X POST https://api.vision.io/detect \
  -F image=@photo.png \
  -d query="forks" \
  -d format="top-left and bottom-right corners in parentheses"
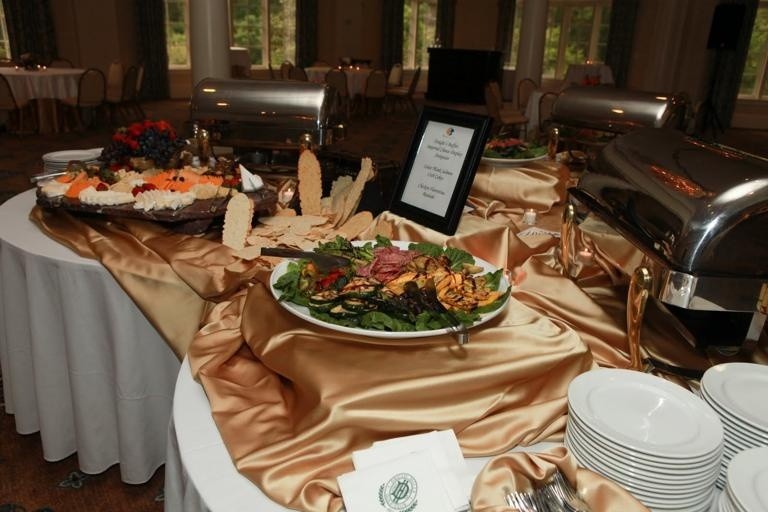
top-left (505, 468), bottom-right (593, 512)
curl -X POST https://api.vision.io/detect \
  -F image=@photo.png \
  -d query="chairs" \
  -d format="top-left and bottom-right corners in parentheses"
top-left (268, 60), bottom-right (309, 81)
top-left (361, 70), bottom-right (387, 119)
top-left (49, 58), bottom-right (73, 67)
top-left (313, 60), bottom-right (329, 67)
top-left (325, 68), bottom-right (359, 123)
top-left (388, 67), bottom-right (424, 117)
top-left (532, 93), bottom-right (559, 144)
top-left (389, 63), bottom-right (411, 116)
top-left (497, 77), bottom-right (535, 116)
top-left (0, 182), bottom-right (182, 488)
top-left (486, 82), bottom-right (530, 141)
top-left (1, 75), bottom-right (35, 136)
top-left (61, 69), bottom-right (106, 131)
top-left (108, 64), bottom-right (148, 123)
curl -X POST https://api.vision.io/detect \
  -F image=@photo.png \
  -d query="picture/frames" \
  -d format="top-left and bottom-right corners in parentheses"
top-left (389, 103), bottom-right (496, 236)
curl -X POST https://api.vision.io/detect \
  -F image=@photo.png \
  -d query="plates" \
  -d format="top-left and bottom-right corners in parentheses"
top-left (563, 360), bottom-right (767, 512)
top-left (271, 238), bottom-right (512, 339)
top-left (481, 151), bottom-right (547, 164)
top-left (41, 148), bottom-right (101, 176)
